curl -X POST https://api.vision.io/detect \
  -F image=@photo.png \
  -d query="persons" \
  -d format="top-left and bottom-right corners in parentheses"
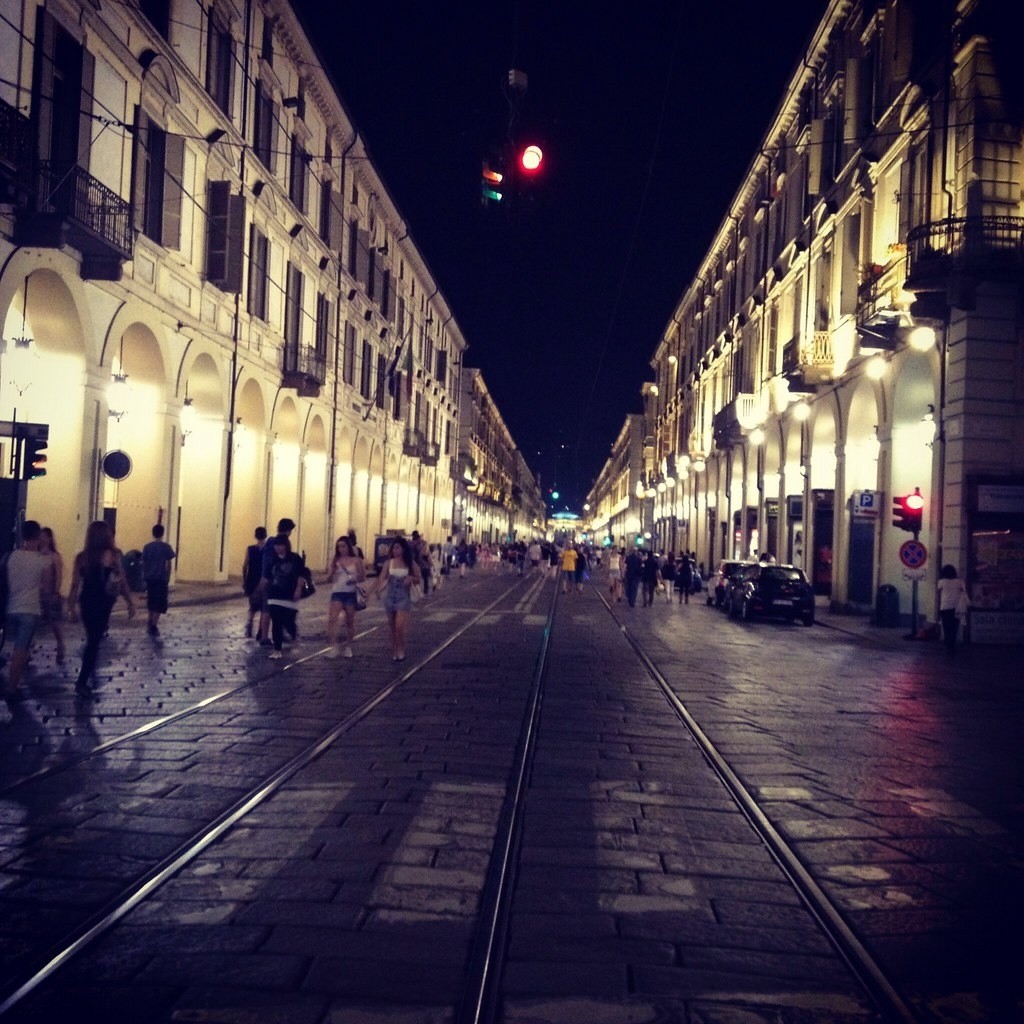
top-left (362, 535), bottom-right (422, 661)
top-left (66, 520), bottom-right (137, 692)
top-left (242, 525), bottom-right (265, 643)
top-left (936, 564), bottom-right (972, 666)
top-left (142, 525), bottom-right (175, 638)
top-left (254, 518), bottom-right (296, 647)
top-left (313, 529), bottom-right (366, 658)
top-left (0, 520), bottom-right (65, 722)
top-left (265, 534), bottom-right (314, 658)
top-left (745, 549), bottom-right (776, 563)
top-left (408, 530), bottom-right (697, 606)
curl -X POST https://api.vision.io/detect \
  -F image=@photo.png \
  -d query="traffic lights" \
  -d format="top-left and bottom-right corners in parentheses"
top-left (892, 497), bottom-right (922, 533)
top-left (478, 151), bottom-right (508, 210)
top-left (510, 139), bottom-right (549, 182)
top-left (24, 435), bottom-right (47, 481)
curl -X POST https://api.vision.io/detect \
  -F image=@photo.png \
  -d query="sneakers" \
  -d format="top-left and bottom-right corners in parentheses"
top-left (268, 652), bottom-right (283, 659)
top-left (289, 647), bottom-right (301, 658)
top-left (325, 647), bottom-right (355, 659)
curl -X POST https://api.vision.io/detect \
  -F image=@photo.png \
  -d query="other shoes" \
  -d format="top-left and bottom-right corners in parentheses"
top-left (3, 690), bottom-right (20, 701)
top-left (460, 574), bottom-right (465, 580)
top-left (147, 625), bottom-right (160, 637)
top-left (75, 686), bottom-right (93, 698)
top-left (55, 644), bottom-right (66, 664)
top-left (28, 655), bottom-right (36, 664)
top-left (393, 648), bottom-right (405, 661)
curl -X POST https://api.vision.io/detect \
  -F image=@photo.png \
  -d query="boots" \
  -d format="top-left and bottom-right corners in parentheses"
top-left (679, 596), bottom-right (689, 604)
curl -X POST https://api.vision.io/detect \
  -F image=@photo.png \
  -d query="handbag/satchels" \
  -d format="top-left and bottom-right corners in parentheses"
top-left (427, 567), bottom-right (437, 588)
top-left (408, 560), bottom-right (426, 605)
top-left (105, 548), bottom-right (122, 599)
top-left (39, 593), bottom-right (61, 622)
top-left (955, 578), bottom-right (970, 618)
top-left (0, 548), bottom-right (15, 625)
top-left (440, 567), bottom-right (449, 575)
top-left (656, 579), bottom-right (664, 595)
top-left (356, 584), bottom-right (367, 611)
top-left (293, 555), bottom-right (315, 599)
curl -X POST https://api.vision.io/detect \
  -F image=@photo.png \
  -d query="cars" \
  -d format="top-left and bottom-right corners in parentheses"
top-left (707, 559), bottom-right (815, 627)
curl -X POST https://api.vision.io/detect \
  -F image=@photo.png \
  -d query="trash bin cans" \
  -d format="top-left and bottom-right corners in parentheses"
top-left (876, 584), bottom-right (898, 626)
top-left (124, 549), bottom-right (146, 594)
